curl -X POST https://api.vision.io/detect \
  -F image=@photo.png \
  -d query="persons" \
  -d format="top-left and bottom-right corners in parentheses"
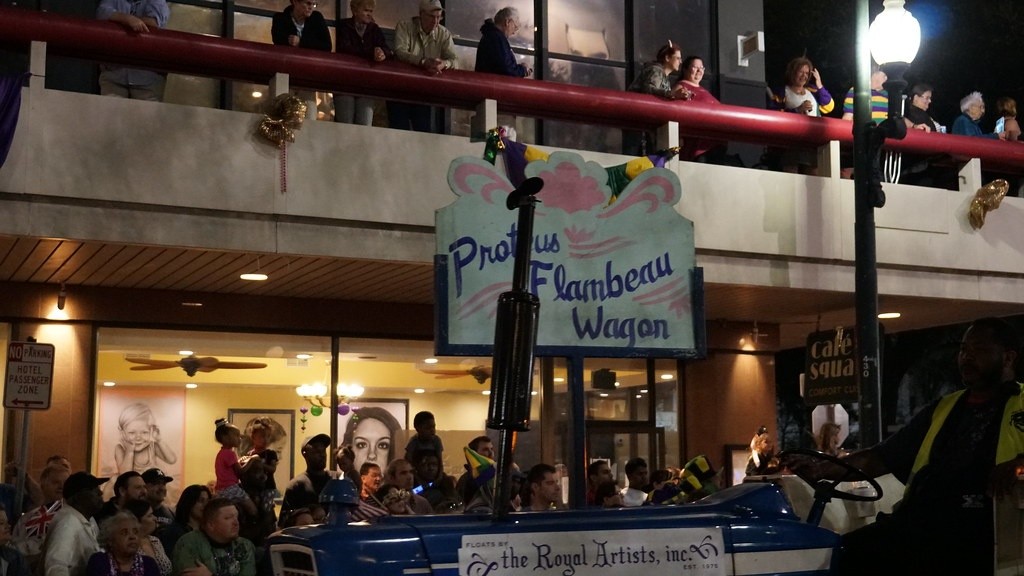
top-left (745, 423), bottom-right (792, 476)
top-left (671, 56), bottom-right (728, 164)
top-left (817, 422), bottom-right (842, 453)
top-left (238, 415), bottom-right (287, 497)
top-left (952, 91), bottom-right (1007, 187)
top-left (765, 48), bottom-right (835, 176)
top-left (386, 0), bottom-right (457, 132)
top-left (96, 0), bottom-right (169, 102)
top-left (277, 410), bottom-right (725, 530)
top-left (334, 1), bottom-right (392, 126)
top-left (341, 407), bottom-right (404, 474)
top-left (0, 453), bottom-right (258, 576)
top-left (215, 417), bottom-right (258, 515)
top-left (900, 81), bottom-right (941, 187)
top-left (994, 96), bottom-right (1024, 198)
top-left (782, 315), bottom-right (1024, 576)
top-left (621, 41), bottom-right (696, 157)
top-left (474, 6), bottom-right (533, 142)
top-left (841, 57), bottom-right (931, 179)
top-left (271, 0), bottom-right (333, 54)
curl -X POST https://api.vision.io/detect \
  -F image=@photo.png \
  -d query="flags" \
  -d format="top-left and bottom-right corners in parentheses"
top-left (25, 498), bottom-right (63, 537)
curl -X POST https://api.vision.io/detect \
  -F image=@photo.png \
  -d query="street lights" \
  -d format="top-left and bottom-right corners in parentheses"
top-left (853, 1), bottom-right (923, 450)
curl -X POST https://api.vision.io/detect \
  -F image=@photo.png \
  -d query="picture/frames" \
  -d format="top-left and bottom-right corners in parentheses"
top-left (332, 398), bottom-right (409, 482)
top-left (228, 409), bottom-right (295, 505)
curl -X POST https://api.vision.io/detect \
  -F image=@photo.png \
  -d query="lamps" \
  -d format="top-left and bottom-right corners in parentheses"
top-left (295, 383), bottom-right (365, 409)
top-left (751, 320), bottom-right (759, 343)
top-left (58, 285), bottom-right (67, 310)
top-left (591, 368), bottom-right (617, 391)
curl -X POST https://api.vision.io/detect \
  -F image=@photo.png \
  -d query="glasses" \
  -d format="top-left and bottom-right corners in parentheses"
top-left (511, 19), bottom-right (519, 32)
top-left (303, 1), bottom-right (319, 8)
top-left (920, 94), bottom-right (931, 101)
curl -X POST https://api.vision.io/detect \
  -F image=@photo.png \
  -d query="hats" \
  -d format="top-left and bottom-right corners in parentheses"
top-left (301, 433), bottom-right (332, 453)
top-left (419, 0), bottom-right (445, 13)
top-left (63, 471), bottom-right (109, 499)
top-left (141, 469), bottom-right (173, 483)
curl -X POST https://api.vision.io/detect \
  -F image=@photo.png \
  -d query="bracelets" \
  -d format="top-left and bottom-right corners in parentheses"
top-left (421, 57), bottom-right (427, 68)
top-left (444, 60), bottom-right (448, 69)
top-left (912, 123), bottom-right (916, 128)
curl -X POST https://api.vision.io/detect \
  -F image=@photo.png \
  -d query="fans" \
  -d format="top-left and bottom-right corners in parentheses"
top-left (125, 354), bottom-right (268, 377)
top-left (420, 363), bottom-right (492, 384)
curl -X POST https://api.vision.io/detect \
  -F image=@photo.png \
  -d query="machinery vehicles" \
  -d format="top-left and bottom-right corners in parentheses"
top-left (259, 445), bottom-right (902, 576)
